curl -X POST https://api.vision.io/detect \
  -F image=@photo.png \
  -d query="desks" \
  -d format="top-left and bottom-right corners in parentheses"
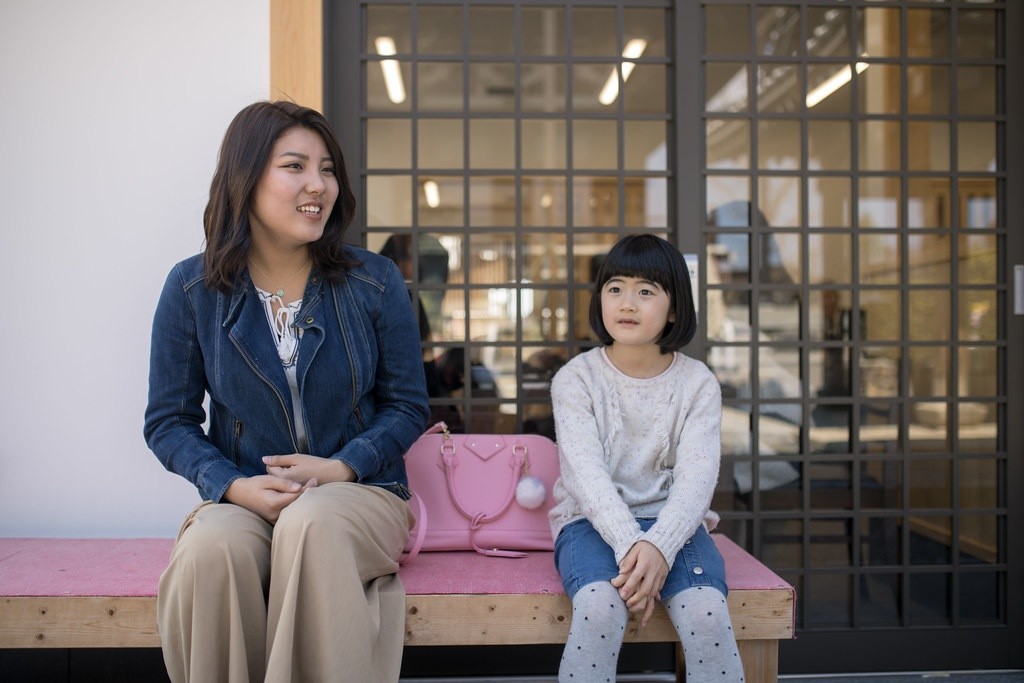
top-left (712, 410), bottom-right (998, 605)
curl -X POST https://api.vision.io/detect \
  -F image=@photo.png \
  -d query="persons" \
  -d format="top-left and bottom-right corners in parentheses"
top-left (705, 201), bottom-right (811, 569)
top-left (143, 101), bottom-right (431, 683)
top-left (548, 232), bottom-right (745, 683)
top-left (381, 232), bottom-right (479, 435)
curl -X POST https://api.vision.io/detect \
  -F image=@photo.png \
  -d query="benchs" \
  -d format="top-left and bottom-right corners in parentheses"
top-left (0, 537), bottom-right (796, 683)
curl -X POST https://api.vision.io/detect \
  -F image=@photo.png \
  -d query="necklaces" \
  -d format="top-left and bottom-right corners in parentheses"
top-left (247, 254), bottom-right (313, 298)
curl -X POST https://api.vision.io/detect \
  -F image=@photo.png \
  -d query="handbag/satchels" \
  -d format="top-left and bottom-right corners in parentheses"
top-left (393, 421), bottom-right (560, 565)
